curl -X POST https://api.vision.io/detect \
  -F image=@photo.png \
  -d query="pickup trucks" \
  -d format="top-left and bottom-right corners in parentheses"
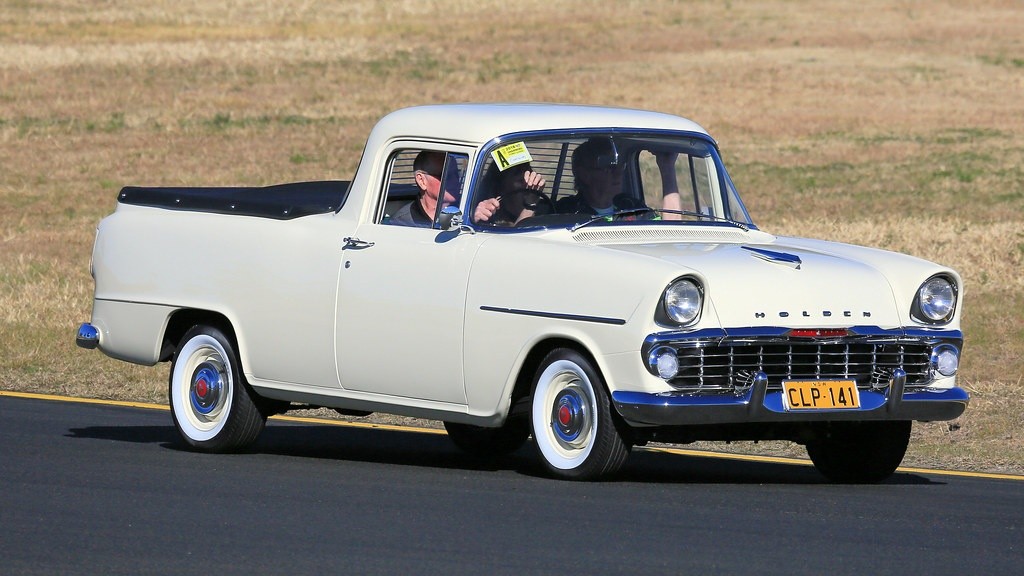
top-left (74, 102), bottom-right (972, 484)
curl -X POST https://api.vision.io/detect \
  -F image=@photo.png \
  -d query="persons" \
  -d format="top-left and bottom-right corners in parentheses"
top-left (554, 137), bottom-right (684, 220)
top-left (385, 149), bottom-right (564, 229)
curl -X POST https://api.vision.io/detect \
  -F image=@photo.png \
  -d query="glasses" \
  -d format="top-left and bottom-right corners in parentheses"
top-left (585, 162), bottom-right (626, 174)
top-left (426, 169), bottom-right (464, 181)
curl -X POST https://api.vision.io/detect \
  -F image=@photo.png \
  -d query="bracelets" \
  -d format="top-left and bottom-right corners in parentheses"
top-left (523, 199), bottom-right (536, 210)
